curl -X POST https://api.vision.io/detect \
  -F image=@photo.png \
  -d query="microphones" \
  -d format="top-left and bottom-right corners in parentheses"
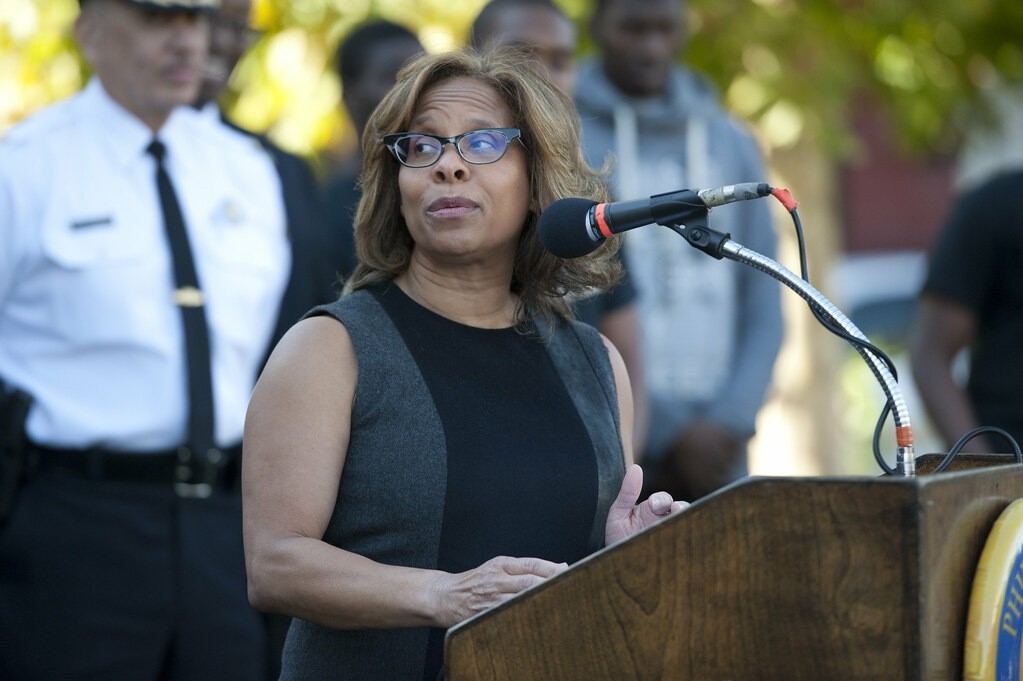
top-left (538, 183), bottom-right (770, 259)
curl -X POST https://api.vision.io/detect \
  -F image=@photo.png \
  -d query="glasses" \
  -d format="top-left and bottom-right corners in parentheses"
top-left (383, 127), bottom-right (531, 168)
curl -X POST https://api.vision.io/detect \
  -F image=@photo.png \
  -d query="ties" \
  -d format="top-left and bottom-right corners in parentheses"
top-left (146, 139), bottom-right (215, 469)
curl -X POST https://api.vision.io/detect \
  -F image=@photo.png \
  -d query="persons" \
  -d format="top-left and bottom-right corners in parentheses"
top-left (240, 47), bottom-right (692, 679)
top-left (0, 0), bottom-right (1022, 679)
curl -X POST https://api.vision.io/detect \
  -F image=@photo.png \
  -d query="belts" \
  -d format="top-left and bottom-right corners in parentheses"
top-left (39, 450), bottom-right (247, 484)
